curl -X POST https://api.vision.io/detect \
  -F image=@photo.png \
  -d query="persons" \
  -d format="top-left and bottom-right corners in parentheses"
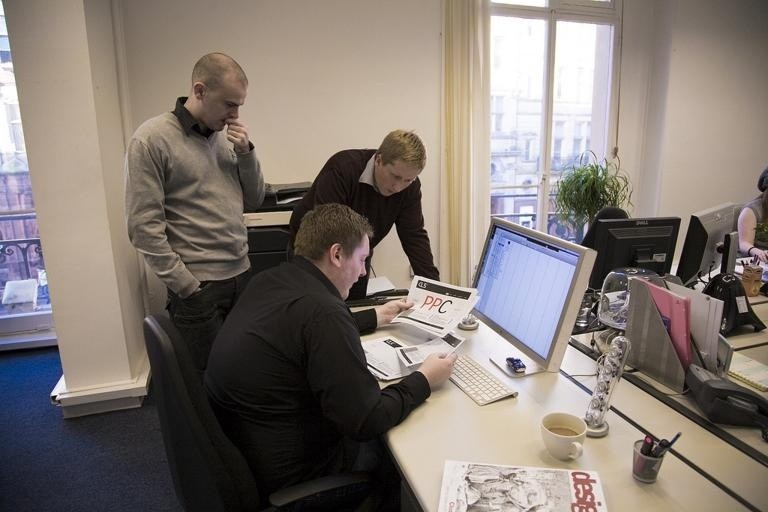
top-left (203, 202), bottom-right (457, 512)
top-left (737, 168), bottom-right (768, 263)
top-left (125, 52), bottom-right (266, 386)
top-left (287, 130), bottom-right (440, 302)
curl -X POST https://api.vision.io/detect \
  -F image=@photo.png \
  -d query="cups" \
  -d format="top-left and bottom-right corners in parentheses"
top-left (540, 412), bottom-right (587, 463)
top-left (630, 441), bottom-right (664, 483)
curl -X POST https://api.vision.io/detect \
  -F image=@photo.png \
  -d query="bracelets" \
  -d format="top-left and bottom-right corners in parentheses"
top-left (748, 247), bottom-right (757, 256)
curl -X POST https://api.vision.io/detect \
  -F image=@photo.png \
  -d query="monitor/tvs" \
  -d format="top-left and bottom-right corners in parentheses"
top-left (590, 216), bottom-right (681, 290)
top-left (469, 215), bottom-right (599, 378)
top-left (676, 201), bottom-right (739, 287)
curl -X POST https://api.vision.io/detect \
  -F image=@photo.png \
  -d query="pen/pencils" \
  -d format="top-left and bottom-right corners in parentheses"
top-left (669, 432), bottom-right (682, 447)
top-left (741, 253), bottom-right (760, 266)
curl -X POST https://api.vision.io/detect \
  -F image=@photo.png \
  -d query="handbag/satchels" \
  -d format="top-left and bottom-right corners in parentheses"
top-left (571, 287), bottom-right (608, 336)
top-left (701, 272), bottom-right (766, 334)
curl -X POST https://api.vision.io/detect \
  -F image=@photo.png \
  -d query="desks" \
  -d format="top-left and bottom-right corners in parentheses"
top-left (345, 250), bottom-right (768, 512)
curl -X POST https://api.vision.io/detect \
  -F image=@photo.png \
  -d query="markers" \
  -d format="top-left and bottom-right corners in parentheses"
top-left (642, 435), bottom-right (655, 456)
top-left (654, 438), bottom-right (670, 456)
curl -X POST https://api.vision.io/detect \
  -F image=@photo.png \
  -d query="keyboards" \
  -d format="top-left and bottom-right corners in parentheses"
top-left (449, 353), bottom-right (519, 407)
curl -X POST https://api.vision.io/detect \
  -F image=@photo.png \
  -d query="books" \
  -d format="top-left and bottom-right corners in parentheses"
top-left (438, 459), bottom-right (607, 512)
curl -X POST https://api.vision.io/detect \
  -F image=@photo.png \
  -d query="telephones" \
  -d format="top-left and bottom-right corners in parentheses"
top-left (684, 362), bottom-right (767, 428)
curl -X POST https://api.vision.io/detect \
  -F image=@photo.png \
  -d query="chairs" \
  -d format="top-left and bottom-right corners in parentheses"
top-left (142, 313), bottom-right (378, 511)
top-left (733, 204), bottom-right (745, 260)
